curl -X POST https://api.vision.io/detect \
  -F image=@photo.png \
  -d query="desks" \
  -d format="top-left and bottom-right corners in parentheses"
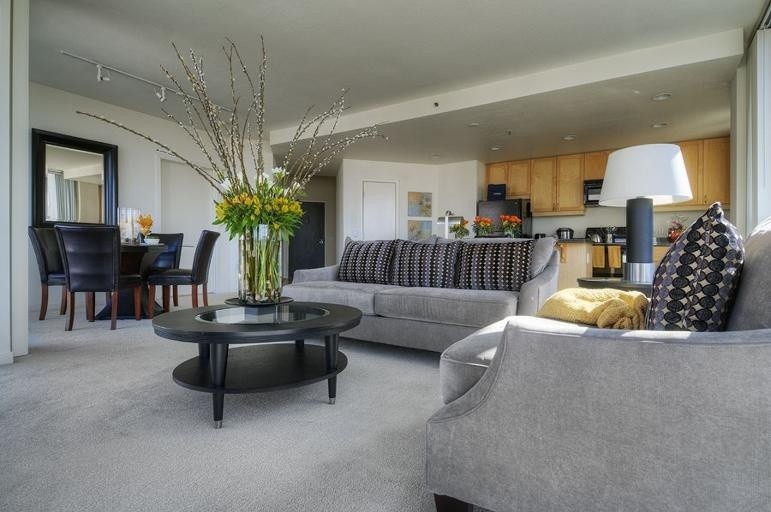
top-left (580, 273), bottom-right (665, 302)
top-left (94, 242), bottom-right (178, 321)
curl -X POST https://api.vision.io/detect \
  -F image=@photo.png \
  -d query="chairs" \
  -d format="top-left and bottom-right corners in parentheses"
top-left (139, 231), bottom-right (186, 309)
top-left (145, 229), bottom-right (220, 322)
top-left (26, 222), bottom-right (96, 324)
top-left (52, 220), bottom-right (145, 332)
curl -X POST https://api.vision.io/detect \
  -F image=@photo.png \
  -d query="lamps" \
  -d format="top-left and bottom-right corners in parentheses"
top-left (597, 143), bottom-right (695, 282)
top-left (435, 208), bottom-right (463, 240)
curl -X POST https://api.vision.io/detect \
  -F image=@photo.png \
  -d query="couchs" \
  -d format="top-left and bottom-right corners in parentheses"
top-left (283, 235), bottom-right (560, 358)
top-left (419, 216), bottom-right (771, 512)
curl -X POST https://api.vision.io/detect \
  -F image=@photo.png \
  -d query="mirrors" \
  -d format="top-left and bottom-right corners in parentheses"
top-left (31, 126), bottom-right (120, 228)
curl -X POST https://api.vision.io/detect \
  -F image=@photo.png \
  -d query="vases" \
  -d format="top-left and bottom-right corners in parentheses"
top-left (478, 229), bottom-right (488, 236)
top-left (234, 222), bottom-right (285, 300)
top-left (502, 227), bottom-right (515, 237)
top-left (454, 233), bottom-right (464, 238)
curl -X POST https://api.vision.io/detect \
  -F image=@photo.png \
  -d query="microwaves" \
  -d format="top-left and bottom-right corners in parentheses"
top-left (583, 181), bottom-right (603, 207)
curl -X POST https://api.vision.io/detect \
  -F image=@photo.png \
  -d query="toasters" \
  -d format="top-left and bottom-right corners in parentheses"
top-left (556, 228), bottom-right (574, 239)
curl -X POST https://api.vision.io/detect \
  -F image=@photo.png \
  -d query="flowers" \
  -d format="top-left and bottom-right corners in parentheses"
top-left (449, 218), bottom-right (468, 235)
top-left (470, 215), bottom-right (498, 233)
top-left (498, 212), bottom-right (520, 235)
top-left (67, 32), bottom-right (391, 298)
top-left (136, 214), bottom-right (154, 236)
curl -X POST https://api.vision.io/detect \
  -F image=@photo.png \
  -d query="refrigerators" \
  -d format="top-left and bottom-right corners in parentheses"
top-left (477, 198), bottom-right (533, 237)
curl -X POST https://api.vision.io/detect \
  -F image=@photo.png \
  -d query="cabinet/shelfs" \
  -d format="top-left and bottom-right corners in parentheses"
top-left (700, 134), bottom-right (730, 212)
top-left (531, 151), bottom-right (585, 217)
top-left (585, 148), bottom-right (607, 179)
top-left (650, 138), bottom-right (700, 212)
top-left (485, 158), bottom-right (531, 199)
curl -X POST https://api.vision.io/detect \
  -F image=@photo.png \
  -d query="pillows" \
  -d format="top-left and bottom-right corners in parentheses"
top-left (335, 234), bottom-right (393, 283)
top-left (642, 202), bottom-right (745, 335)
top-left (390, 239), bottom-right (460, 294)
top-left (458, 238), bottom-right (536, 290)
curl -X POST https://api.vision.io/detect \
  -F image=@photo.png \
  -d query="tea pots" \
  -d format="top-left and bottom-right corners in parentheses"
top-left (587, 233), bottom-right (602, 243)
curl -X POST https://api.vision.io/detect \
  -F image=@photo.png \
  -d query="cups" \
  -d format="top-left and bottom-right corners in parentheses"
top-left (605, 233), bottom-right (615, 244)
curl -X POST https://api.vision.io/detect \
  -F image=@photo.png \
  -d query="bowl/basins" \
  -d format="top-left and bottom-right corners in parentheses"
top-left (144, 239), bottom-right (161, 245)
